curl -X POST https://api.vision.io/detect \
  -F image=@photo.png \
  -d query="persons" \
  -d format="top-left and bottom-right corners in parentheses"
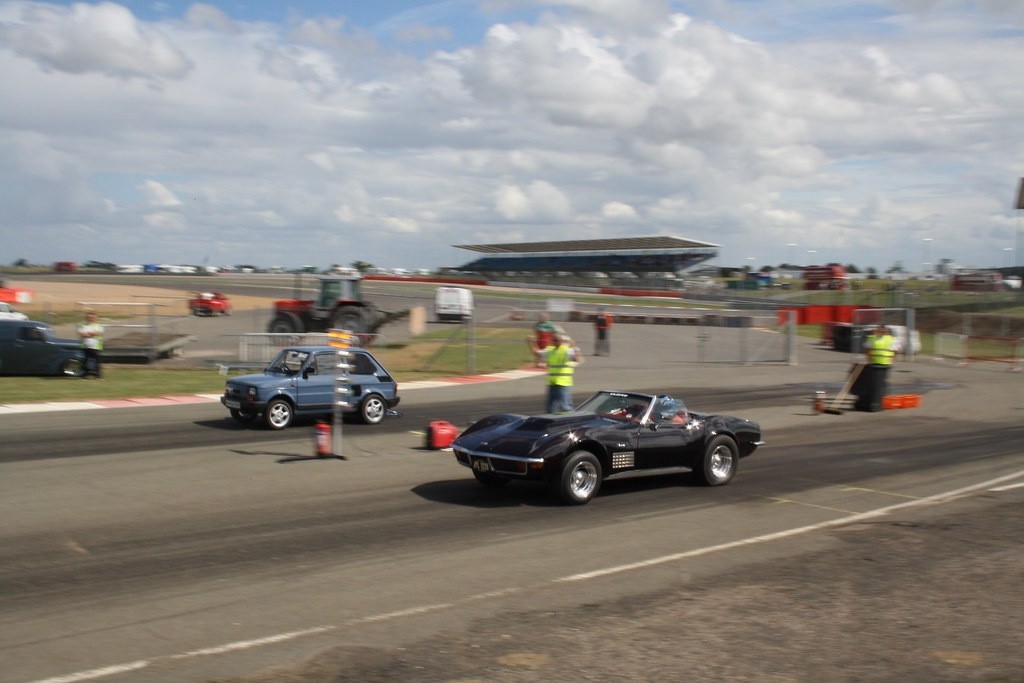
top-left (649, 394), bottom-right (684, 427)
top-left (532, 311), bottom-right (562, 367)
top-left (592, 306), bottom-right (612, 358)
top-left (526, 332), bottom-right (584, 415)
top-left (77, 312), bottom-right (105, 379)
top-left (861, 325), bottom-right (903, 397)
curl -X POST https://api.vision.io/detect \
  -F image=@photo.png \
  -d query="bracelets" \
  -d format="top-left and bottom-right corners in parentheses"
top-left (574, 347), bottom-right (580, 352)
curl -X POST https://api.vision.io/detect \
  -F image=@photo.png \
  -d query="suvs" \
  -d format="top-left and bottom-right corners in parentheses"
top-left (1002, 274), bottom-right (1022, 292)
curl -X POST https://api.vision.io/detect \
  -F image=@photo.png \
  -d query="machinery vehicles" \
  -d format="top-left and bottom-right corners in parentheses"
top-left (268, 274), bottom-right (409, 346)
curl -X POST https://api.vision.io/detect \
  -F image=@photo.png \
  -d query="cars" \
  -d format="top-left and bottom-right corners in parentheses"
top-left (0, 318), bottom-right (89, 378)
top-left (220, 346), bottom-right (400, 430)
top-left (0, 301), bottom-right (30, 321)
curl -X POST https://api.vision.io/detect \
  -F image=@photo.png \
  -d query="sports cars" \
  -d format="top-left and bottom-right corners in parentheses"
top-left (451, 390), bottom-right (765, 505)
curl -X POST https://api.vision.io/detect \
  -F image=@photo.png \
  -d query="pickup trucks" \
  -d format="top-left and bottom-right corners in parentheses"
top-left (189, 292), bottom-right (234, 317)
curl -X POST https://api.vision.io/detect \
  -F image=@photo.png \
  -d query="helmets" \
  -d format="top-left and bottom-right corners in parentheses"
top-left (654, 395), bottom-right (677, 417)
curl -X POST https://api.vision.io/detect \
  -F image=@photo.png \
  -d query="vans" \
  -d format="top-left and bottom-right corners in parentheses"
top-left (434, 287), bottom-right (473, 323)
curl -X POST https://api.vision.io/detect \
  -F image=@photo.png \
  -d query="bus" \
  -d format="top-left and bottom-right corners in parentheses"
top-left (952, 270), bottom-right (1002, 292)
top-left (55, 260), bottom-right (79, 274)
top-left (805, 265), bottom-right (847, 291)
